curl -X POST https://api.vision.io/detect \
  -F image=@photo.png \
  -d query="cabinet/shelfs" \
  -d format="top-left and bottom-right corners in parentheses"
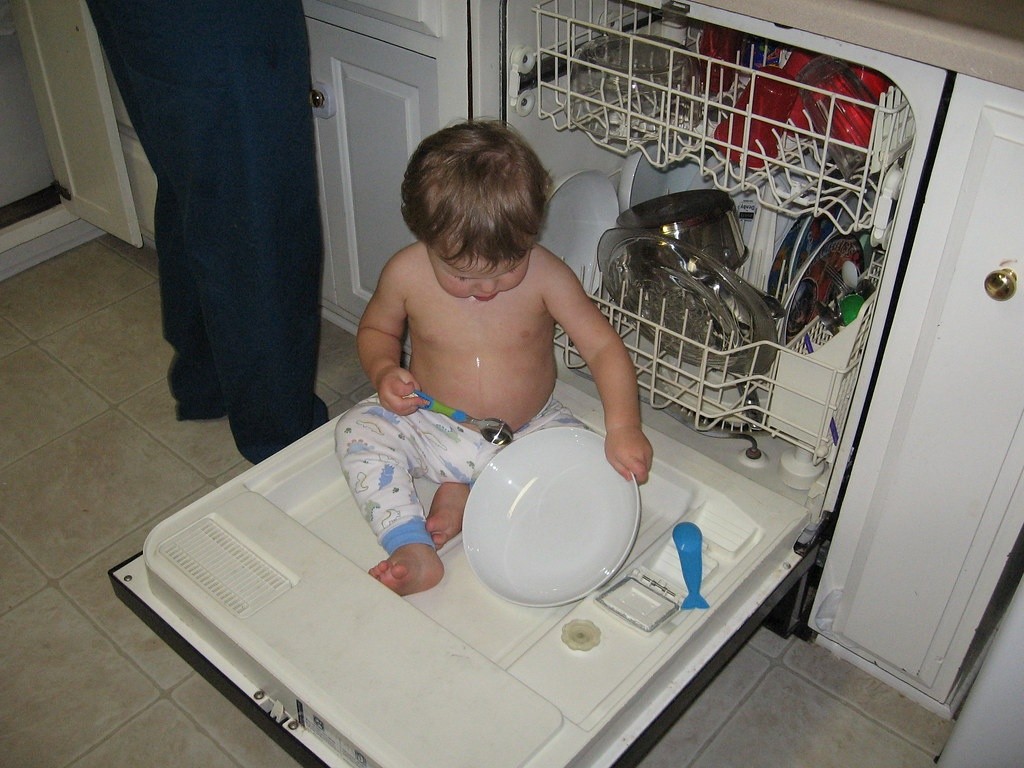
top-left (90, 0), bottom-right (1024, 768)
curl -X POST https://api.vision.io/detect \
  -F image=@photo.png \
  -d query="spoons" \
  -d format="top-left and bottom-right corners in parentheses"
top-left (814, 261), bottom-right (876, 335)
top-left (414, 389), bottom-right (514, 449)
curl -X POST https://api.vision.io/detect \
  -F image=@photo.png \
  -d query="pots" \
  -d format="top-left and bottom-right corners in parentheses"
top-left (616, 188), bottom-right (766, 429)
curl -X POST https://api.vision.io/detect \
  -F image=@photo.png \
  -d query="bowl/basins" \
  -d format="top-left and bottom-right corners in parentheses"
top-left (564, 32), bottom-right (702, 136)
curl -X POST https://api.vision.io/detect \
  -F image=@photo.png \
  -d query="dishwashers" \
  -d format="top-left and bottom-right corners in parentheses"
top-left (106, 0), bottom-right (954, 768)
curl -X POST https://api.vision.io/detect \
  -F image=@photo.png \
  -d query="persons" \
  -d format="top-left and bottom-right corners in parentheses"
top-left (334, 120), bottom-right (653, 595)
top-left (87, 0), bottom-right (330, 467)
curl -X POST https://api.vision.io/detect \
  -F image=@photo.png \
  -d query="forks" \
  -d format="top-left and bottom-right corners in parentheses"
top-left (823, 265), bottom-right (853, 296)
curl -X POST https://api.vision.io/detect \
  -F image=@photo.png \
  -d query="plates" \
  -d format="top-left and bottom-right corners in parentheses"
top-left (461, 425), bottom-right (642, 610)
top-left (618, 137), bottom-right (701, 216)
top-left (695, 153), bottom-right (866, 344)
top-left (540, 167), bottom-right (621, 296)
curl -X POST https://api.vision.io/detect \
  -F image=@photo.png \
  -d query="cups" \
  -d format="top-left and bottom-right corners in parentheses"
top-left (714, 50), bottom-right (896, 181)
top-left (697, 22), bottom-right (741, 93)
